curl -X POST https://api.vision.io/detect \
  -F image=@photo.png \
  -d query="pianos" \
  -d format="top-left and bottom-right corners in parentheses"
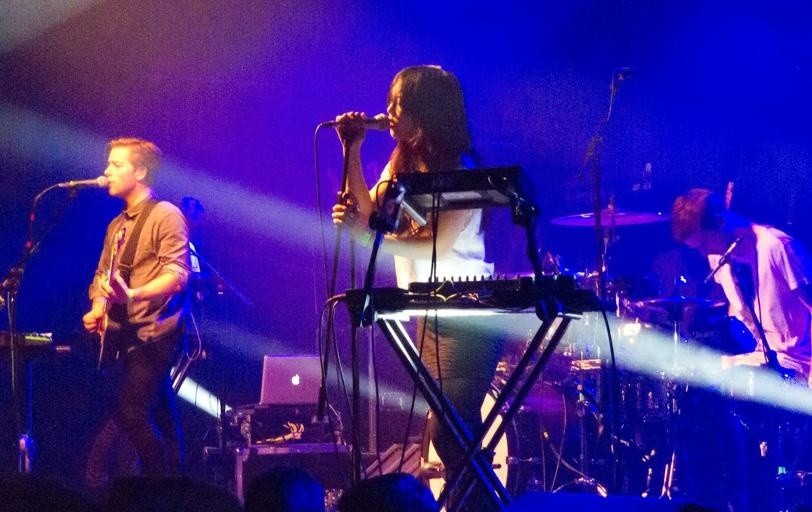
top-left (349, 270), bottom-right (613, 323)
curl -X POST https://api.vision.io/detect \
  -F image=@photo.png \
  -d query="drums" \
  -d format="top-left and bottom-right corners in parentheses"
top-left (620, 324), bottom-right (725, 422)
top-left (421, 354), bottom-right (588, 512)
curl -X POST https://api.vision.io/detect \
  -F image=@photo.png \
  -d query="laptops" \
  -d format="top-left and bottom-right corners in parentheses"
top-left (239, 353), bottom-right (323, 408)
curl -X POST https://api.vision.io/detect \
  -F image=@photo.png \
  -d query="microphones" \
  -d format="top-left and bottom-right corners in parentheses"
top-left (62, 176), bottom-right (110, 190)
top-left (323, 113), bottom-right (393, 132)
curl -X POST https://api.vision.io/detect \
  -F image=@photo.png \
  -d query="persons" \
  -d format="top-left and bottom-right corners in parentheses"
top-left (181, 197), bottom-right (211, 304)
top-left (653, 245), bottom-right (697, 340)
top-left (1, 473), bottom-right (442, 512)
top-left (331, 64), bottom-right (509, 510)
top-left (501, 220), bottom-right (555, 272)
top-left (672, 187), bottom-right (812, 388)
top-left (81, 136), bottom-right (191, 491)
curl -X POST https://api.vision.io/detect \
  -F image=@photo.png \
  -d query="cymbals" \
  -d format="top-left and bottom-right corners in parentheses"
top-left (632, 298), bottom-right (724, 312)
top-left (551, 208), bottom-right (669, 228)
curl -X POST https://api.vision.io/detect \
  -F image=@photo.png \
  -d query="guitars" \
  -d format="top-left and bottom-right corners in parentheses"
top-left (96, 227), bottom-right (125, 371)
